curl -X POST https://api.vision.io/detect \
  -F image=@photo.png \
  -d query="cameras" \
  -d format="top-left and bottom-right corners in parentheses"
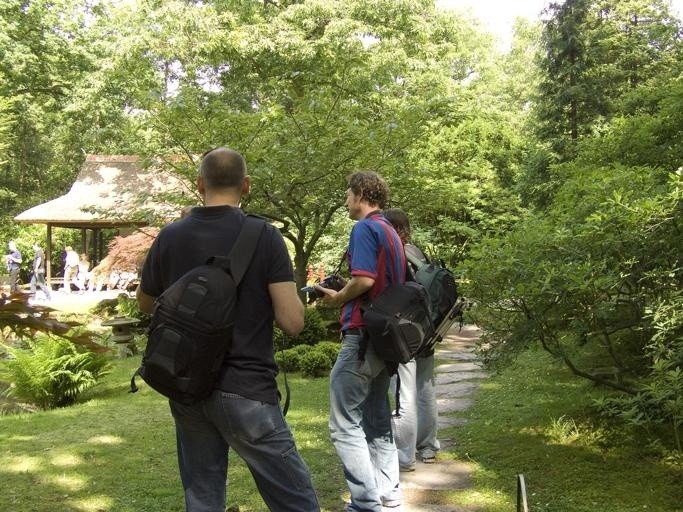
top-left (301, 276), bottom-right (344, 304)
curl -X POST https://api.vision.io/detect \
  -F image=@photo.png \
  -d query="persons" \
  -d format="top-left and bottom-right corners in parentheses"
top-left (134, 147), bottom-right (320, 512)
top-left (29, 243), bottom-right (50, 301)
top-left (78, 252), bottom-right (90, 291)
top-left (4, 241), bottom-right (22, 294)
top-left (378, 207), bottom-right (441, 472)
top-left (315, 169), bottom-right (406, 512)
top-left (63, 246), bottom-right (84, 295)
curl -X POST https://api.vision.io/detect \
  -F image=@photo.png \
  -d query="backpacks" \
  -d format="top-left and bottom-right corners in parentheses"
top-left (355, 283), bottom-right (434, 377)
top-left (128, 212), bottom-right (265, 407)
top-left (403, 244), bottom-right (458, 332)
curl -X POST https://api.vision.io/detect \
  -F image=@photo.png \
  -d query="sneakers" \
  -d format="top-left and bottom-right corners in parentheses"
top-left (344, 500), bottom-right (365, 511)
